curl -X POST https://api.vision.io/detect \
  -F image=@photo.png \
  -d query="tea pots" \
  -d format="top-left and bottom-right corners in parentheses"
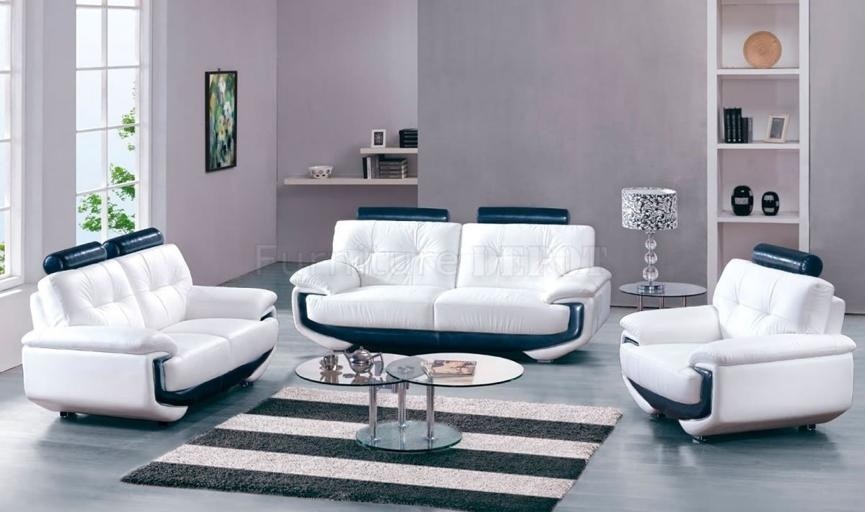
top-left (343, 345), bottom-right (385, 376)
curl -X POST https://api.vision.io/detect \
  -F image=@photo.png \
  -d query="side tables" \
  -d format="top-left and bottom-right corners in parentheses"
top-left (619, 282), bottom-right (707, 311)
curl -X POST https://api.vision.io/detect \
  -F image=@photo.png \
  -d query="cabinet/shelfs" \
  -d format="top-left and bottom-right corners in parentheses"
top-left (282, 147), bottom-right (418, 186)
top-left (706, 0), bottom-right (810, 306)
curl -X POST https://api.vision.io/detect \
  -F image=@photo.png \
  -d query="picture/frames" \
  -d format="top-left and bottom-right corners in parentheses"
top-left (371, 130), bottom-right (386, 147)
top-left (766, 114), bottom-right (789, 142)
top-left (205, 71), bottom-right (238, 172)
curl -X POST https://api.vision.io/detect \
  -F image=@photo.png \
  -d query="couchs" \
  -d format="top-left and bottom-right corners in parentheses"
top-left (291, 205), bottom-right (612, 363)
top-left (22, 227), bottom-right (279, 423)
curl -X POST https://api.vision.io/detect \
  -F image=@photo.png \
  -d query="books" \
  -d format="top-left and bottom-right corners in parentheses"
top-left (398, 127), bottom-right (418, 148)
top-left (363, 156), bottom-right (409, 180)
top-left (421, 358), bottom-right (477, 378)
top-left (722, 106), bottom-right (749, 144)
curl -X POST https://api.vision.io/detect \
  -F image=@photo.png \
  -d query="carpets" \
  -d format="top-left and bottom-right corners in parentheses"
top-left (121, 385), bottom-right (623, 512)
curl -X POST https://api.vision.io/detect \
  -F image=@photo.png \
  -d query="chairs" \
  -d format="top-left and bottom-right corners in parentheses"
top-left (619, 243), bottom-right (857, 444)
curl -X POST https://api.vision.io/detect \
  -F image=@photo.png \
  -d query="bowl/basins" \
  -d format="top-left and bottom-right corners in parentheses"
top-left (307, 165), bottom-right (335, 179)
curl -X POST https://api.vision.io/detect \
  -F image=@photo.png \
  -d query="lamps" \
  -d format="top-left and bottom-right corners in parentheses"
top-left (621, 187), bottom-right (678, 291)
